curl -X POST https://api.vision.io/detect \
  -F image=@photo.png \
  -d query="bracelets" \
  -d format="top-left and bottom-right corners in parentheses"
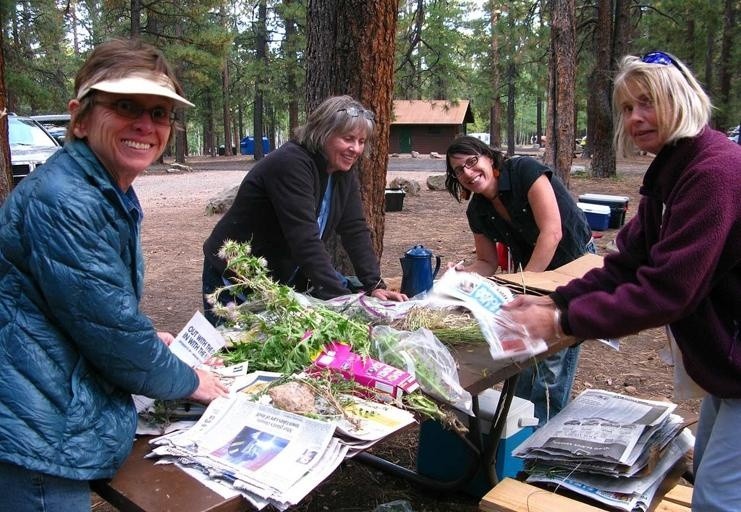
top-left (553, 302), bottom-right (561, 340)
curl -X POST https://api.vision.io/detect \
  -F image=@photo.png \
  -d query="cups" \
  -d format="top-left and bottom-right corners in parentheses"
top-left (399, 245), bottom-right (443, 298)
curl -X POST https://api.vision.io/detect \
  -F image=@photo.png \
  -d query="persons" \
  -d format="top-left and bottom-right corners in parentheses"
top-left (199, 95), bottom-right (409, 327)
top-left (2, 39), bottom-right (234, 512)
top-left (438, 135), bottom-right (598, 434)
top-left (497, 51), bottom-right (741, 512)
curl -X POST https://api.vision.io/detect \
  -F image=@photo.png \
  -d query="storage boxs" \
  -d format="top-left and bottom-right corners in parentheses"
top-left (579, 193), bottom-right (630, 229)
top-left (386, 186), bottom-right (407, 213)
top-left (576, 202), bottom-right (611, 231)
top-left (478, 476), bottom-right (694, 511)
top-left (417, 389), bottom-right (540, 492)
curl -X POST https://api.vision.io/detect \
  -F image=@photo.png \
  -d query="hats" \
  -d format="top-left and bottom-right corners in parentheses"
top-left (77, 68), bottom-right (196, 107)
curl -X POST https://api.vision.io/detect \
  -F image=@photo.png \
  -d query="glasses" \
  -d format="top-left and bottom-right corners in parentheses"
top-left (450, 154), bottom-right (483, 178)
top-left (337, 108), bottom-right (377, 122)
top-left (93, 98), bottom-right (177, 126)
top-left (637, 52), bottom-right (683, 65)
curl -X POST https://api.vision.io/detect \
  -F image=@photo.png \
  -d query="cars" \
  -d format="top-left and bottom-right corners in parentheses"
top-left (726, 125), bottom-right (741, 144)
top-left (6, 115), bottom-right (71, 187)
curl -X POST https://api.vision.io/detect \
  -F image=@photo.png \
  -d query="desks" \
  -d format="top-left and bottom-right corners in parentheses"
top-left (90, 319), bottom-right (574, 511)
